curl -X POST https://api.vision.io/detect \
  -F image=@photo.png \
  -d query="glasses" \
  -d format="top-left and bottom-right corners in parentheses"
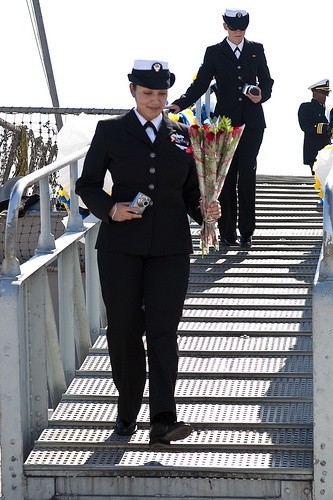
top-left (228, 25), bottom-right (246, 31)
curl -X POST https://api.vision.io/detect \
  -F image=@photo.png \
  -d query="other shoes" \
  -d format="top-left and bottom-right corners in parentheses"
top-left (114, 412), bottom-right (138, 435)
top-left (220, 233), bottom-right (240, 246)
top-left (239, 234), bottom-right (252, 246)
top-left (150, 418), bottom-right (192, 445)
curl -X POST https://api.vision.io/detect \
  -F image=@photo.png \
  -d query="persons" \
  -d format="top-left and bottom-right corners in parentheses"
top-left (166, 8), bottom-right (274, 248)
top-left (75, 60), bottom-right (219, 449)
top-left (298, 79), bottom-right (333, 175)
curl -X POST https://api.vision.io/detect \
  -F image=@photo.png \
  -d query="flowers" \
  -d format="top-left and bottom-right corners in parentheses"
top-left (189, 115), bottom-right (246, 257)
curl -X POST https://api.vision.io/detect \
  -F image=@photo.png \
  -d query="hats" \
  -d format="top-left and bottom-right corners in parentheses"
top-left (222, 9), bottom-right (249, 26)
top-left (127, 59), bottom-right (176, 90)
top-left (307, 78), bottom-right (332, 92)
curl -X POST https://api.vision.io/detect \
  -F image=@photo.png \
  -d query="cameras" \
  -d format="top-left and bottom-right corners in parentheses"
top-left (242, 84), bottom-right (260, 97)
top-left (130, 192), bottom-right (151, 216)
